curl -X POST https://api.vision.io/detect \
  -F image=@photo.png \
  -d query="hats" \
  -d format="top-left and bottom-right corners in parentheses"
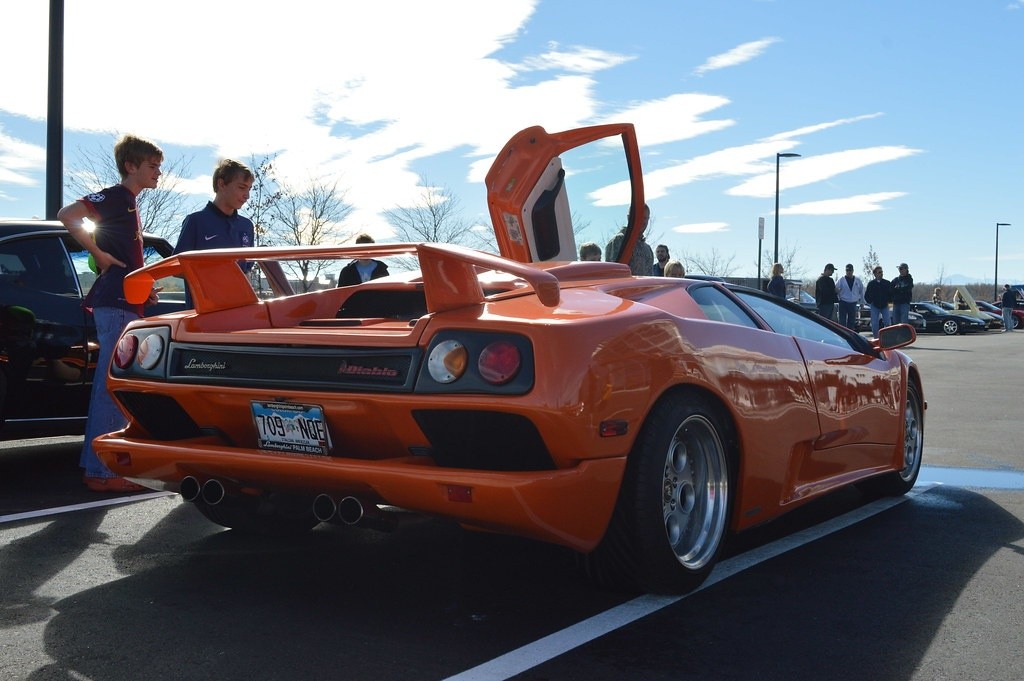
top-left (846, 264), bottom-right (853, 270)
top-left (826, 264), bottom-right (837, 270)
top-left (896, 263), bottom-right (908, 269)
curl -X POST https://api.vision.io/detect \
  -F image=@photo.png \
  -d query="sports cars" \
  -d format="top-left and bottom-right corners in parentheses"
top-left (93, 123), bottom-right (928, 590)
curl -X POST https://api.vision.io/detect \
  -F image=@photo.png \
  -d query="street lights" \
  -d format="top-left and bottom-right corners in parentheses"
top-left (994, 223), bottom-right (1011, 302)
top-left (774, 153), bottom-right (801, 263)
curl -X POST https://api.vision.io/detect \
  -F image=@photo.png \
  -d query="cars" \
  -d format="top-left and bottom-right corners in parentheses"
top-left (992, 299), bottom-right (1024, 311)
top-left (966, 301), bottom-right (1024, 329)
top-left (910, 303), bottom-right (986, 335)
top-left (879, 308), bottom-right (927, 330)
top-left (922, 301), bottom-right (1005, 329)
top-left (0, 219), bottom-right (185, 440)
top-left (933, 286), bottom-right (995, 329)
top-left (786, 289), bottom-right (839, 312)
top-left (855, 309), bottom-right (883, 330)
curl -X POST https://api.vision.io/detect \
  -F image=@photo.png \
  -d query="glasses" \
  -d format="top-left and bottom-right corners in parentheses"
top-left (878, 271), bottom-right (883, 273)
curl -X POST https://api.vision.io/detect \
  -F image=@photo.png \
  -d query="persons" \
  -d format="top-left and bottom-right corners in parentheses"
top-left (172, 158), bottom-right (254, 310)
top-left (815, 263), bottom-right (839, 318)
top-left (767, 263), bottom-right (786, 299)
top-left (835, 264), bottom-right (864, 330)
top-left (579, 241), bottom-right (602, 261)
top-left (664, 259), bottom-right (685, 278)
top-left (652, 245), bottom-right (669, 276)
top-left (831, 368), bottom-right (899, 414)
top-left (338, 234), bottom-right (389, 288)
top-left (864, 266), bottom-right (892, 339)
top-left (932, 287), bottom-right (942, 308)
top-left (952, 290), bottom-right (962, 310)
top-left (605, 201), bottom-right (653, 277)
top-left (1001, 284), bottom-right (1016, 332)
top-left (890, 263), bottom-right (914, 349)
top-left (57, 135), bottom-right (164, 493)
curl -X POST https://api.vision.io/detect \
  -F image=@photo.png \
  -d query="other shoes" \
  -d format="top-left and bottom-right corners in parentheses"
top-left (84, 475), bottom-right (146, 491)
